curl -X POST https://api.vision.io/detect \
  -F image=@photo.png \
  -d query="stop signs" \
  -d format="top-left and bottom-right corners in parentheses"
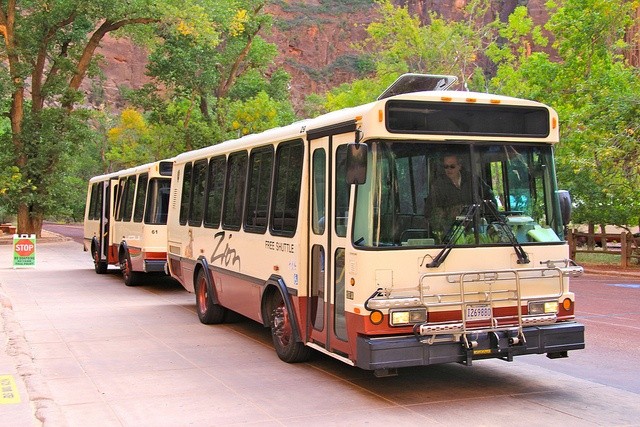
top-left (13, 233), bottom-right (36, 266)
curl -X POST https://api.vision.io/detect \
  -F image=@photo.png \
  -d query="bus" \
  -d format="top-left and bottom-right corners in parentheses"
top-left (83, 159), bottom-right (173, 285)
top-left (166, 73), bottom-right (584, 370)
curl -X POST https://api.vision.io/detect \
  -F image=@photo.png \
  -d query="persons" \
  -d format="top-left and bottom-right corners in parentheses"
top-left (425, 152), bottom-right (498, 213)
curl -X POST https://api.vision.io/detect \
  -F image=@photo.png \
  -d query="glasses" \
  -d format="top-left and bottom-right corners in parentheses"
top-left (444, 164), bottom-right (456, 169)
